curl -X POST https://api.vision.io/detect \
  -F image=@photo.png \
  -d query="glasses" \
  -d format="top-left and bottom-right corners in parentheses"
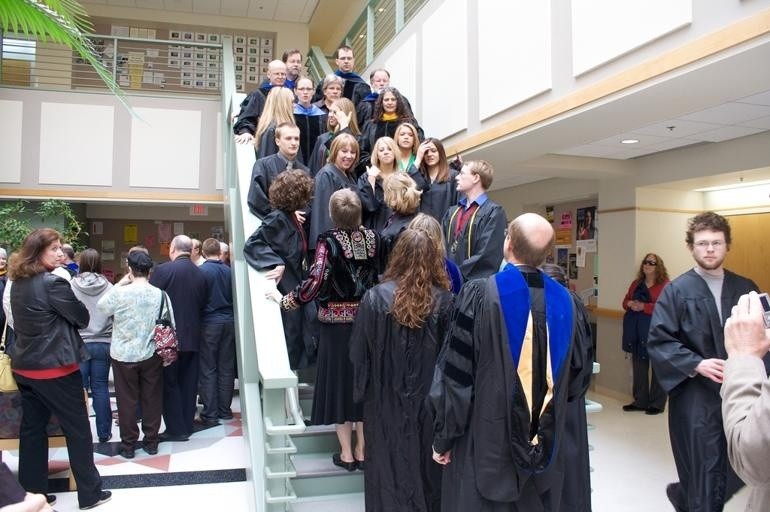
top-left (642, 260), bottom-right (656, 266)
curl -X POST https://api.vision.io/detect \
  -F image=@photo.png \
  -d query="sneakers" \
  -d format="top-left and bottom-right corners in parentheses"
top-left (46, 495), bottom-right (58, 506)
top-left (158, 409), bottom-right (234, 440)
top-left (80, 491), bottom-right (112, 510)
top-left (623, 404), bottom-right (648, 411)
top-left (645, 407), bottom-right (662, 414)
top-left (101, 434), bottom-right (157, 456)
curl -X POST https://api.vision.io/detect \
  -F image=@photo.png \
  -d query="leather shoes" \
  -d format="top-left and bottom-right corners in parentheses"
top-left (333, 453), bottom-right (364, 471)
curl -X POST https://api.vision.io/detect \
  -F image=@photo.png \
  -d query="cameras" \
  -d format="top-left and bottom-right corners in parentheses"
top-left (758, 293), bottom-right (770, 328)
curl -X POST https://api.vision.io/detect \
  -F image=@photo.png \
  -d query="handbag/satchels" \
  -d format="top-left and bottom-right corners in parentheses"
top-left (154, 319), bottom-right (180, 367)
top-left (0, 349), bottom-right (19, 393)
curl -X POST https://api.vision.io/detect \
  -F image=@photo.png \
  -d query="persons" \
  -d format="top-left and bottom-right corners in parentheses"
top-left (231, 45), bottom-right (509, 472)
top-left (717, 290), bottom-right (770, 512)
top-left (643, 210), bottom-right (763, 512)
top-left (577, 209), bottom-right (594, 239)
top-left (427, 212), bottom-right (596, 511)
top-left (619, 252), bottom-right (670, 415)
top-left (0, 228), bottom-right (236, 512)
top-left (347, 228), bottom-right (457, 512)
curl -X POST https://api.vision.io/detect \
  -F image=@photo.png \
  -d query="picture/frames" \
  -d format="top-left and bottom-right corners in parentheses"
top-left (168, 30), bottom-right (273, 92)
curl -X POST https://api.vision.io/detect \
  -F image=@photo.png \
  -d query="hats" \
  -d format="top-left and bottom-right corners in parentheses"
top-left (128, 250), bottom-right (153, 272)
